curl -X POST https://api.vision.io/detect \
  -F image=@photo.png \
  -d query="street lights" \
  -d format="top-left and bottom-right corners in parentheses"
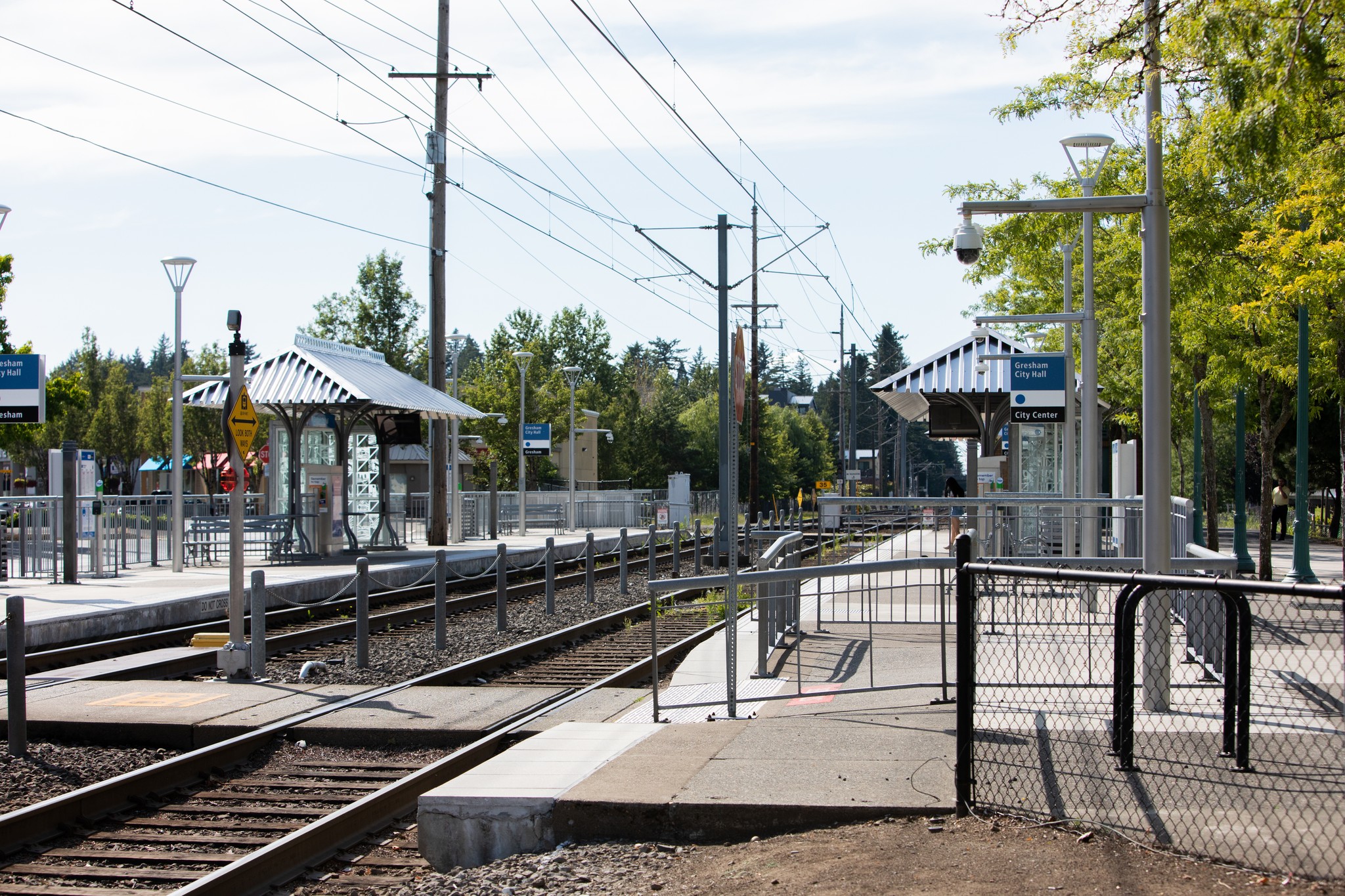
top-left (563, 366), bottom-right (583, 532)
top-left (444, 333), bottom-right (468, 544)
top-left (1059, 135), bottom-right (1116, 558)
top-left (159, 256), bottom-right (196, 574)
top-left (512, 350), bottom-right (535, 535)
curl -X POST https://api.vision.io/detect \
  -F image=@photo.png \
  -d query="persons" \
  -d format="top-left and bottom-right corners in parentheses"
top-left (1270, 477), bottom-right (1291, 542)
top-left (943, 476), bottom-right (965, 550)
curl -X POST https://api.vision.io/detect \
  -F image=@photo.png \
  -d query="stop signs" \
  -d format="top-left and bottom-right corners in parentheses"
top-left (258, 445), bottom-right (270, 463)
top-left (731, 324), bottom-right (748, 426)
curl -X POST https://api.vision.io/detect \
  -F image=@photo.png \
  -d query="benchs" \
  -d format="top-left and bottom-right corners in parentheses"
top-left (1001, 524), bottom-right (1059, 599)
top-left (182, 514), bottom-right (297, 568)
top-left (497, 502), bottom-right (566, 537)
top-left (947, 522), bottom-right (1002, 595)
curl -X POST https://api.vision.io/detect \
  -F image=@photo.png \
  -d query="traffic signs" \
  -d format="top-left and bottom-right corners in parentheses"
top-left (1009, 356), bottom-right (1065, 423)
top-left (230, 384), bottom-right (259, 461)
